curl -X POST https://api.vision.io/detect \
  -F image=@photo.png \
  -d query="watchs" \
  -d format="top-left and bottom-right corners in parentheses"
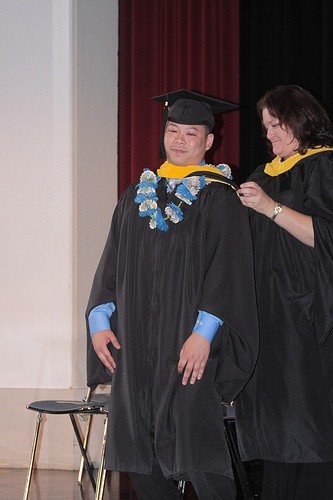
top-left (271, 203), bottom-right (282, 221)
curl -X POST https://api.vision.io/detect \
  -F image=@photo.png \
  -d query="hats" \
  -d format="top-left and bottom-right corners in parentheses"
top-left (150, 89), bottom-right (241, 161)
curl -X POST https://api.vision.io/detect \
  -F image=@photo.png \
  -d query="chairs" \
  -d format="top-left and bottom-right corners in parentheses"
top-left (23, 335), bottom-right (115, 500)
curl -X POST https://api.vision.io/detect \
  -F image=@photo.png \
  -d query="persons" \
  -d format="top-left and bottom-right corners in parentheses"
top-left (235, 84), bottom-right (333, 500)
top-left (85, 88), bottom-right (260, 500)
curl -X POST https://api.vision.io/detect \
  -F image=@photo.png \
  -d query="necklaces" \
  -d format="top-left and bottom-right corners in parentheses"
top-left (135, 164), bottom-right (233, 231)
